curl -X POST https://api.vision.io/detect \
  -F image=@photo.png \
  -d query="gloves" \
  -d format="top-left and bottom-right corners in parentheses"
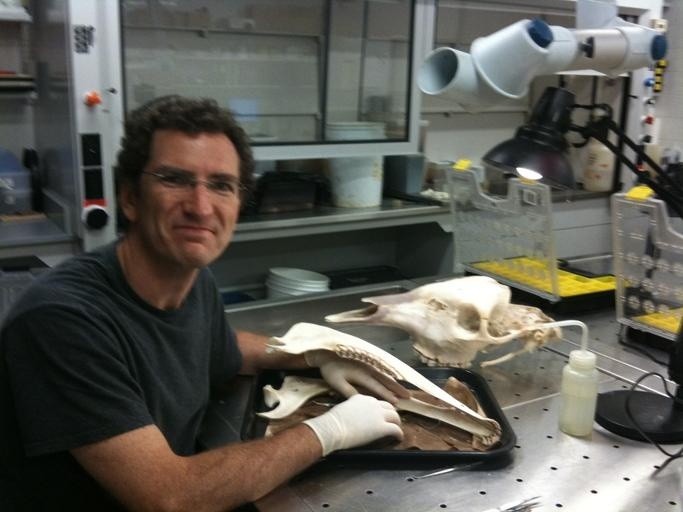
top-left (304, 350), bottom-right (411, 404)
top-left (302, 393), bottom-right (405, 458)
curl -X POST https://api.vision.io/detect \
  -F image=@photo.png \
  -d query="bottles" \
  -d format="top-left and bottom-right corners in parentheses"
top-left (555, 350), bottom-right (600, 439)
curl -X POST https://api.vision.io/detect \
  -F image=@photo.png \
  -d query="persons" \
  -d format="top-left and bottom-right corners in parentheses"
top-left (1, 93), bottom-right (413, 511)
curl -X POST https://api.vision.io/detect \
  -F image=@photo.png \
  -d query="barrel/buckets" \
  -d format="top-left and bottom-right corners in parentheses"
top-left (583, 137), bottom-right (615, 192)
top-left (324, 121), bottom-right (385, 208)
top-left (265, 266), bottom-right (330, 299)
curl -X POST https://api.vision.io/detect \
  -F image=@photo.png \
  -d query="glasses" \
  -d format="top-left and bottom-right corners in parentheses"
top-left (144, 168), bottom-right (248, 199)
